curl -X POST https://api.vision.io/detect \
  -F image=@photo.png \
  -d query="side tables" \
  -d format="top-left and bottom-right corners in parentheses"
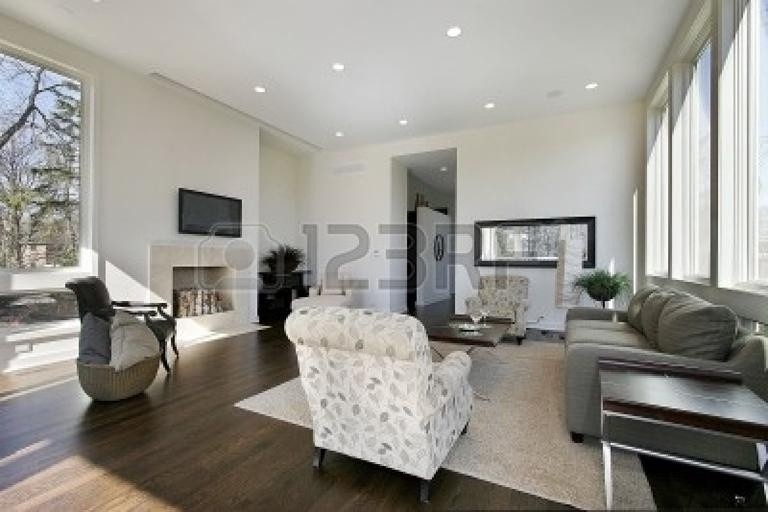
top-left (598, 359), bottom-right (768, 511)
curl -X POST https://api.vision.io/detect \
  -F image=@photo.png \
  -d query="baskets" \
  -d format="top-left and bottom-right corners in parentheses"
top-left (76, 347), bottom-right (163, 403)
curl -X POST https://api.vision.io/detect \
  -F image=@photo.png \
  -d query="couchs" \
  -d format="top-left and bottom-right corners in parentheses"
top-left (564, 287), bottom-right (768, 445)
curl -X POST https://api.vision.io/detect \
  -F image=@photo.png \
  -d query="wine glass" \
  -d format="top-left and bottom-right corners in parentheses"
top-left (471, 308), bottom-right (489, 336)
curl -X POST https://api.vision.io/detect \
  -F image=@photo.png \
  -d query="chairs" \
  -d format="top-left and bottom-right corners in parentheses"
top-left (64, 276), bottom-right (180, 372)
top-left (285, 305), bottom-right (473, 504)
top-left (466, 275), bottom-right (529, 344)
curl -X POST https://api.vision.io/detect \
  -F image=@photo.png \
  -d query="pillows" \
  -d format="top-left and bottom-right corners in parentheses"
top-left (627, 286), bottom-right (738, 360)
top-left (76, 310), bottom-right (112, 366)
top-left (109, 308), bottom-right (161, 372)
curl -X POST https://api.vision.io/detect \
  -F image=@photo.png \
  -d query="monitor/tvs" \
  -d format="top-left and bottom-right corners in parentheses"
top-left (179, 187), bottom-right (242, 238)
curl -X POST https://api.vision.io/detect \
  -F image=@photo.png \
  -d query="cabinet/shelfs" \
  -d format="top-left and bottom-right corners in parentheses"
top-left (258, 271), bottom-right (309, 327)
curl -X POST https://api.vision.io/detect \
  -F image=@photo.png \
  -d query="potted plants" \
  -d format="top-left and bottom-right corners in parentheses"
top-left (264, 244), bottom-right (304, 274)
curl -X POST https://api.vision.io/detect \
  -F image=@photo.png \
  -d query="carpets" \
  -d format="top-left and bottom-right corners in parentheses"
top-left (235, 339), bottom-right (658, 511)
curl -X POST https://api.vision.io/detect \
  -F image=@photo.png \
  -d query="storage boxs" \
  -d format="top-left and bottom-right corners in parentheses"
top-left (75, 355), bottom-right (159, 402)
